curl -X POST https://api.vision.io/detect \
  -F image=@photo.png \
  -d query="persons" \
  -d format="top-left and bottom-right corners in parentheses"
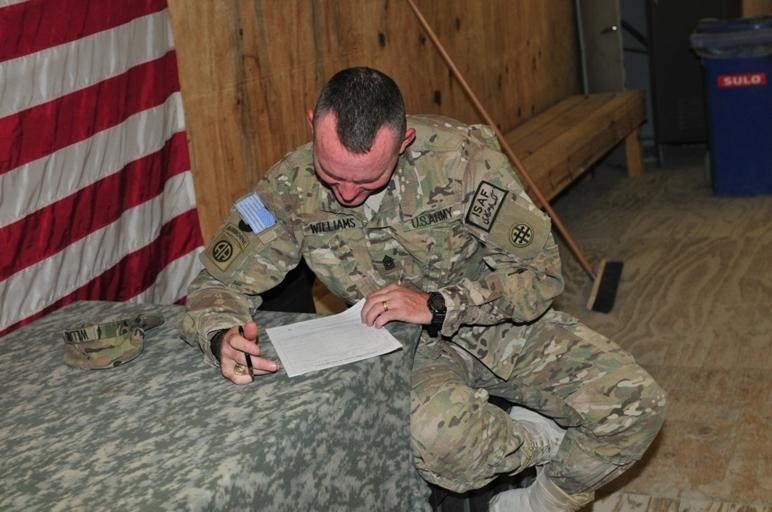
top-left (177, 66), bottom-right (669, 510)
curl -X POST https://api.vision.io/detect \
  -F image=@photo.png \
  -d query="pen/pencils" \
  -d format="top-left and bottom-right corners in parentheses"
top-left (238, 325), bottom-right (255, 382)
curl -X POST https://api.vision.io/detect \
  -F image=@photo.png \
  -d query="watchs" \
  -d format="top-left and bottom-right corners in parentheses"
top-left (422, 290), bottom-right (446, 338)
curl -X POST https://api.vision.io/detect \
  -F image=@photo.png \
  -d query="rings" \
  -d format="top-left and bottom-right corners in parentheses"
top-left (232, 362), bottom-right (247, 377)
top-left (382, 300), bottom-right (390, 313)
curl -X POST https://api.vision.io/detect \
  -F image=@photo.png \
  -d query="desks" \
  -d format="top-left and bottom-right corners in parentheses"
top-left (1, 299), bottom-right (434, 512)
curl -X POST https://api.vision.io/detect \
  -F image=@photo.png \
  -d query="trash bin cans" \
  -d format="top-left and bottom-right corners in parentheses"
top-left (689, 18), bottom-right (772, 198)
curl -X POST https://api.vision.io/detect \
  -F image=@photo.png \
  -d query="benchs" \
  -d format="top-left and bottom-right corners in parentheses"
top-left (499, 87), bottom-right (650, 208)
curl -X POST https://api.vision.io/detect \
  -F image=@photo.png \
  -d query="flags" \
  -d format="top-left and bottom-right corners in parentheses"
top-left (0, 0), bottom-right (204, 338)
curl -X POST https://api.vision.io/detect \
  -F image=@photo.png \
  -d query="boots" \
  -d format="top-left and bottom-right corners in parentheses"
top-left (487, 466), bottom-right (596, 511)
top-left (508, 406), bottom-right (569, 476)
